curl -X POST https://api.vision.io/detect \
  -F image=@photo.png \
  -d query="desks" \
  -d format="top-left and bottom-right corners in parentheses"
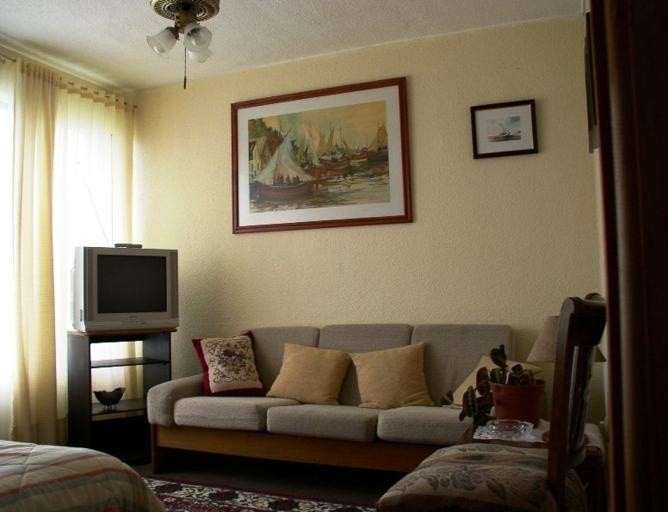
top-left (471, 421), bottom-right (597, 465)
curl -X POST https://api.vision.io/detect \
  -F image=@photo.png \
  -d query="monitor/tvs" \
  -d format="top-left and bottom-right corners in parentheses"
top-left (71, 247), bottom-right (180, 332)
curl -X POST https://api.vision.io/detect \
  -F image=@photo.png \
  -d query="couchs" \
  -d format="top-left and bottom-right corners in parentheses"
top-left (145, 324), bottom-right (519, 475)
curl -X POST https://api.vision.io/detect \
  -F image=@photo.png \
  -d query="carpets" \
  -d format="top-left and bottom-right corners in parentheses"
top-left (142, 475), bottom-right (378, 512)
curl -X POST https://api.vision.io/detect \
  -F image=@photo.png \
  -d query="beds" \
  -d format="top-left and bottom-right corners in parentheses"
top-left (0, 438), bottom-right (164, 512)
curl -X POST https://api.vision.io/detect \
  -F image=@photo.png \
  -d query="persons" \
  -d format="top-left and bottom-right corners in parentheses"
top-left (276, 175), bottom-right (300, 186)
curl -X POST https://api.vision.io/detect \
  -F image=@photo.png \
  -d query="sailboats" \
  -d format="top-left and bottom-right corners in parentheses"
top-left (247, 118), bottom-right (388, 200)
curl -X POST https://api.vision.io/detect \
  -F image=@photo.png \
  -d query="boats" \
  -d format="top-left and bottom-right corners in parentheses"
top-left (489, 129), bottom-right (515, 139)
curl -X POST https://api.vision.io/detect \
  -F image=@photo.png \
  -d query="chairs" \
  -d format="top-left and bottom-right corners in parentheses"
top-left (377, 292), bottom-right (607, 512)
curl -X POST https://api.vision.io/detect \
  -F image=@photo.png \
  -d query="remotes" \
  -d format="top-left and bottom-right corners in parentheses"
top-left (115, 243), bottom-right (142, 248)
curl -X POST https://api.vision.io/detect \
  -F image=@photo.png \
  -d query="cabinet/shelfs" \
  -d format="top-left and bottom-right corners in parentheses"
top-left (68, 332), bottom-right (172, 467)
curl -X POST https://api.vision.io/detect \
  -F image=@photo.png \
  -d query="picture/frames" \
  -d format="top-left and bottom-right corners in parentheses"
top-left (470, 98), bottom-right (539, 159)
top-left (231, 76), bottom-right (413, 234)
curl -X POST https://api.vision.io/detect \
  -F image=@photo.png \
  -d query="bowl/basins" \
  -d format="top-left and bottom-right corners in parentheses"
top-left (93, 389), bottom-right (124, 407)
top-left (484, 418), bottom-right (534, 441)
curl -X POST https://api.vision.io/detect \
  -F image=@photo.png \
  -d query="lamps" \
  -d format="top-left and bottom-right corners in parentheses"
top-left (147, 0), bottom-right (219, 89)
top-left (526, 316), bottom-right (606, 445)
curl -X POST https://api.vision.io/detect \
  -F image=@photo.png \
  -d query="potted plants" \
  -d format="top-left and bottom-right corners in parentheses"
top-left (459, 345), bottom-right (545, 432)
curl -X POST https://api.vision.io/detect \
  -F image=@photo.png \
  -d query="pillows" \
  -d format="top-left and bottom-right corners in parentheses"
top-left (351, 342), bottom-right (437, 408)
top-left (266, 343), bottom-right (352, 406)
top-left (191, 331), bottom-right (266, 397)
top-left (451, 354), bottom-right (544, 416)
